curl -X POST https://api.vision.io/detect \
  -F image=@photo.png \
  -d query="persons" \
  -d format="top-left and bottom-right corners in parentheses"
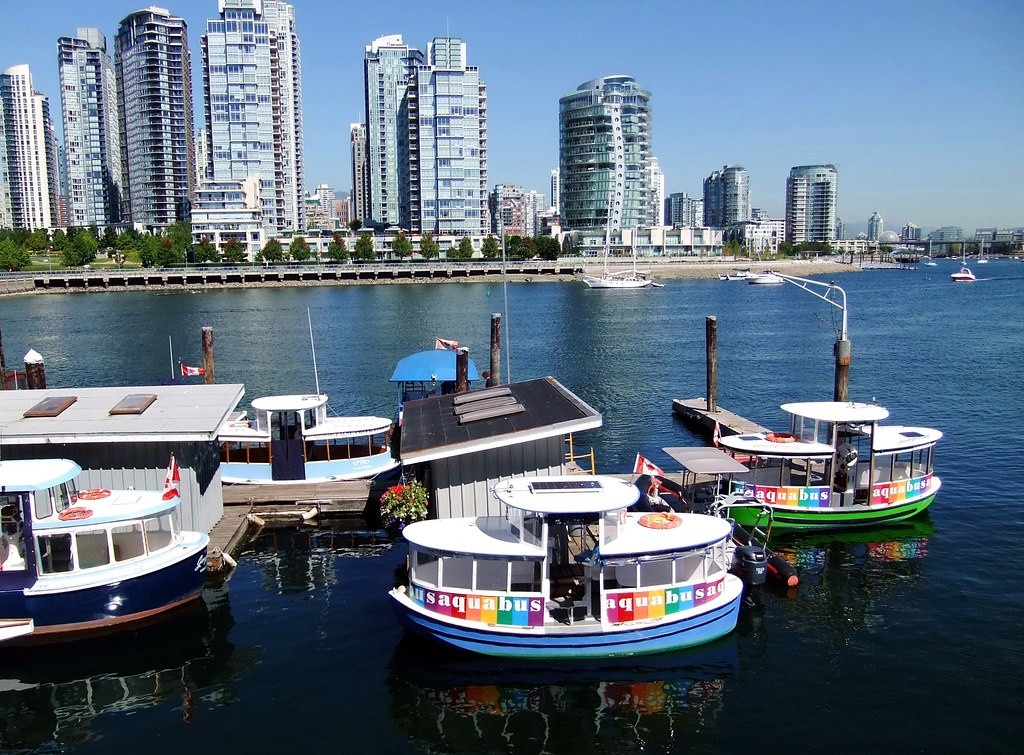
top-left (481, 371), bottom-right (493, 388)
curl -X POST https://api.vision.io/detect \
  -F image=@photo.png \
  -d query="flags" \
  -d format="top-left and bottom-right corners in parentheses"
top-left (713, 421), bottom-right (719, 447)
top-left (633, 455), bottom-right (664, 478)
top-left (163, 457), bottom-right (180, 491)
top-left (435, 339), bottom-right (458, 352)
top-left (181, 365), bottom-right (206, 377)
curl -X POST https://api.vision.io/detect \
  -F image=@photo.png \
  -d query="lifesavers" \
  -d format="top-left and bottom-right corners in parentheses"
top-left (229, 422), bottom-right (253, 428)
top-left (638, 513), bottom-right (683, 529)
top-left (78, 489), bottom-right (111, 500)
top-left (766, 432), bottom-right (799, 443)
top-left (58, 507), bottom-right (93, 521)
top-left (71, 494), bottom-right (77, 502)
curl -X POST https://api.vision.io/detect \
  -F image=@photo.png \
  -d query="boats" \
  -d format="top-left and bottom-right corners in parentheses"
top-left (581, 227), bottom-right (654, 289)
top-left (944, 253), bottom-right (1024, 265)
top-left (389, 336), bottom-right (484, 407)
top-left (717, 266), bottom-right (785, 285)
top-left (924, 258), bottom-right (939, 267)
top-left (665, 272), bottom-right (944, 533)
top-left (387, 474), bottom-right (775, 667)
top-left (0, 307), bottom-right (398, 481)
top-left (950, 266), bottom-right (976, 282)
top-left (0, 457), bottom-right (236, 648)
